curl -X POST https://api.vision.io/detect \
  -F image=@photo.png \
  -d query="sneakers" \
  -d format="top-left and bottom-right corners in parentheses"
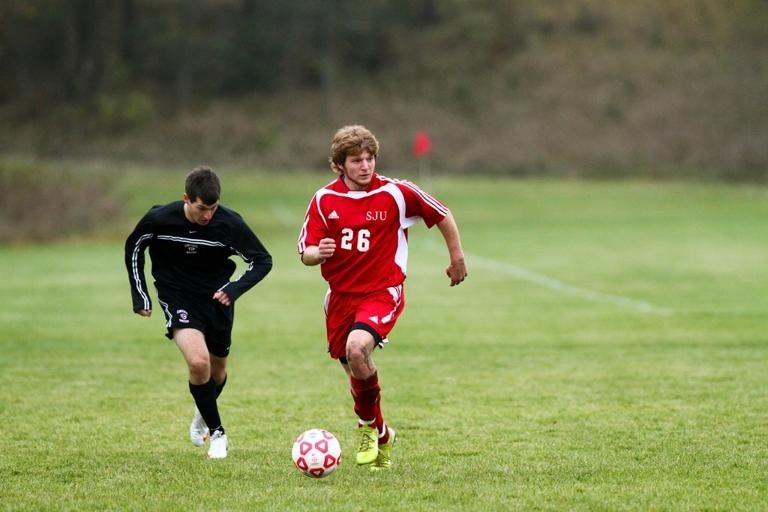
top-left (357, 425), bottom-right (396, 470)
top-left (208, 430), bottom-right (228, 458)
top-left (190, 405), bottom-right (209, 446)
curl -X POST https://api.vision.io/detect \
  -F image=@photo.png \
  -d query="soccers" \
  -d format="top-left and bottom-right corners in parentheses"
top-left (292, 429), bottom-right (341, 478)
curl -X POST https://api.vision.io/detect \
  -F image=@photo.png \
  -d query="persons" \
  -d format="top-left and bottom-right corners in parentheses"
top-left (122, 164), bottom-right (273, 459)
top-left (297, 123), bottom-right (469, 472)
